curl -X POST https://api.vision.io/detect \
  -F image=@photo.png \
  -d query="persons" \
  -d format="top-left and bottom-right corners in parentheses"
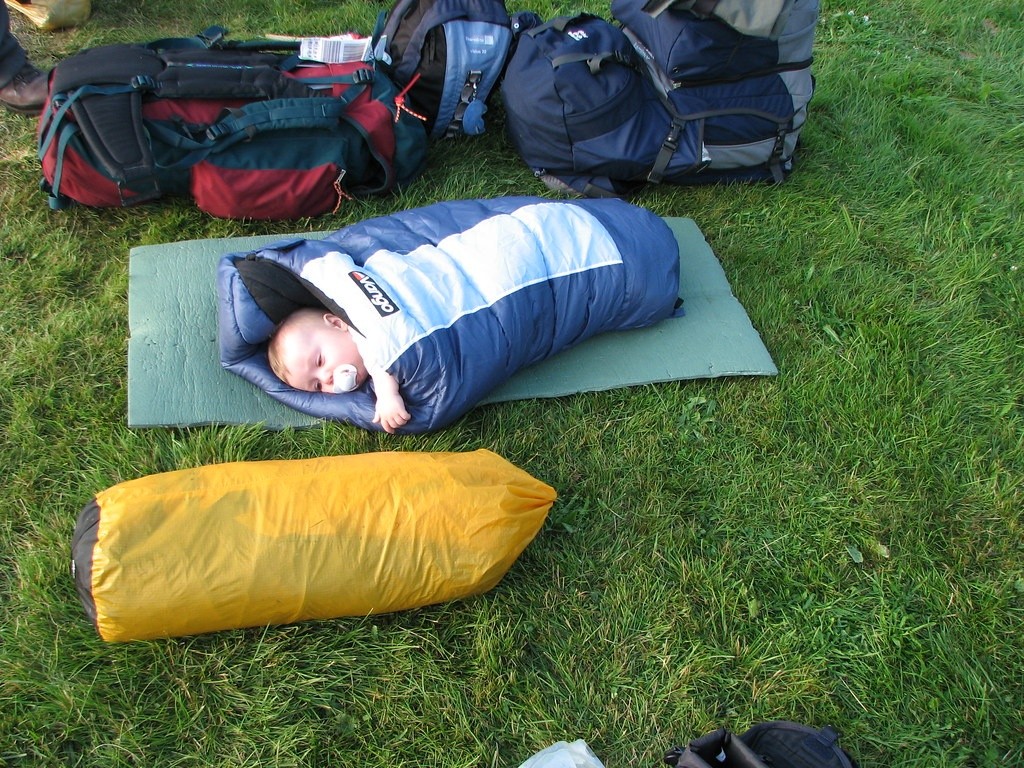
top-left (268, 307), bottom-right (411, 435)
top-left (0, 0), bottom-right (48, 116)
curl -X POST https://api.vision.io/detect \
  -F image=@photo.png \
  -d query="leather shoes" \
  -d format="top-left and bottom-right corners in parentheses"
top-left (0, 61), bottom-right (49, 115)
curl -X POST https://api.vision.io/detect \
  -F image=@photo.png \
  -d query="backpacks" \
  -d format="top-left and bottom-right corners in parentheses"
top-left (38, 28), bottom-right (429, 221)
top-left (373, 0), bottom-right (512, 139)
top-left (502, 0), bottom-right (821, 199)
